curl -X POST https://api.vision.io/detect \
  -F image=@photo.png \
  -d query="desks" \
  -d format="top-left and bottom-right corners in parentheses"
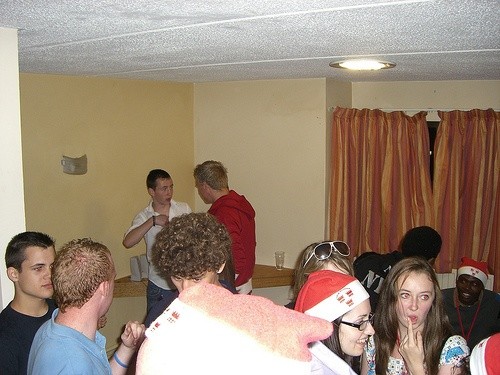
top-left (113, 264), bottom-right (297, 298)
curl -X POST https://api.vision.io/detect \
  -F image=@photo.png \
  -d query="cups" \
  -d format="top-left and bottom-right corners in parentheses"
top-left (275, 254), bottom-right (285, 271)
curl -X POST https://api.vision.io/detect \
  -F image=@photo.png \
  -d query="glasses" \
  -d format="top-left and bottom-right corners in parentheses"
top-left (337, 312), bottom-right (376, 331)
top-left (303, 240), bottom-right (351, 269)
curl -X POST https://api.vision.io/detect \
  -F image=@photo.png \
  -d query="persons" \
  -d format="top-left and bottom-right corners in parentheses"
top-left (286, 226), bottom-right (500, 375)
top-left (27, 238), bottom-right (145, 375)
top-left (192, 159), bottom-right (256, 296)
top-left (0, 231), bottom-right (107, 375)
top-left (146, 213), bottom-right (236, 328)
top-left (124, 170), bottom-right (194, 328)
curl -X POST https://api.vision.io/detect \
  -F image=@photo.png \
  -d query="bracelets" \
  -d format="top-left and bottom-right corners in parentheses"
top-left (153, 216), bottom-right (156, 226)
top-left (114, 352), bottom-right (129, 368)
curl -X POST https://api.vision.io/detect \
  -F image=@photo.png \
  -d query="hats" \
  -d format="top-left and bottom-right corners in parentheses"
top-left (469, 333), bottom-right (500, 375)
top-left (457, 254), bottom-right (489, 289)
top-left (294, 270), bottom-right (372, 323)
top-left (144, 284), bottom-right (334, 375)
